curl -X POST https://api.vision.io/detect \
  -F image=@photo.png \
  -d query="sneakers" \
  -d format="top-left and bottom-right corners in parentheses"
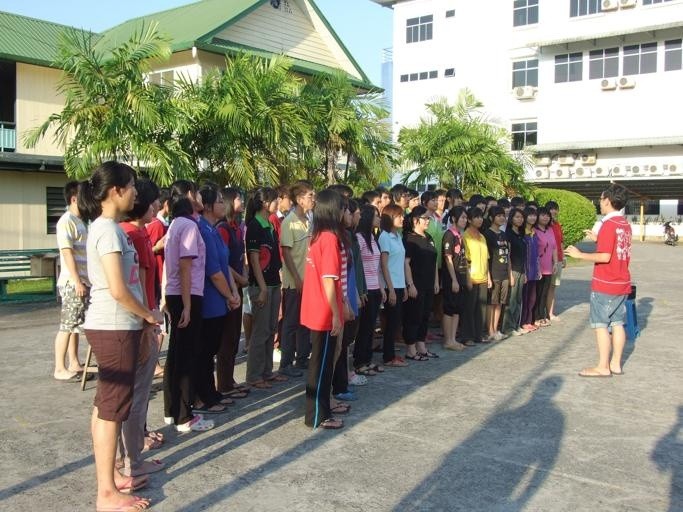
top-left (278, 363), bottom-right (303, 378)
top-left (294, 357), bottom-right (311, 370)
top-left (144, 429), bottom-right (164, 441)
top-left (143, 435), bottom-right (162, 449)
top-left (124, 457), bottom-right (165, 477)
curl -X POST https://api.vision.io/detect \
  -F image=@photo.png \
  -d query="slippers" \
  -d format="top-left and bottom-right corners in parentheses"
top-left (95, 494), bottom-right (152, 512)
top-left (609, 368), bottom-right (623, 376)
top-left (53, 370), bottom-right (94, 383)
top-left (444, 315), bottom-right (561, 351)
top-left (578, 366), bottom-right (612, 378)
top-left (116, 477), bottom-right (148, 492)
top-left (318, 368), bottom-right (367, 429)
top-left (152, 364), bottom-right (164, 379)
top-left (163, 372), bottom-right (289, 433)
top-left (81, 363), bottom-right (98, 372)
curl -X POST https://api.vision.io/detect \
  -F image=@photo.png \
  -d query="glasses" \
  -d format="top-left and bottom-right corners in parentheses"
top-left (419, 216), bottom-right (431, 220)
top-left (598, 197), bottom-right (605, 202)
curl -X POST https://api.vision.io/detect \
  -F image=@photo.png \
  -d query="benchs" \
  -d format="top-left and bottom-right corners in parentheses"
top-left (0, 250), bottom-right (60, 304)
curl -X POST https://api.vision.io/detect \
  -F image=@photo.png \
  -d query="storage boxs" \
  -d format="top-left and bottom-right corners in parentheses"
top-left (28, 254), bottom-right (57, 278)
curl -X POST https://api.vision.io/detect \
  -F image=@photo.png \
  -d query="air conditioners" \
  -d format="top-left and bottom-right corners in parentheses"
top-left (599, 76), bottom-right (616, 88)
top-left (618, 0), bottom-right (637, 7)
top-left (512, 85), bottom-right (533, 99)
top-left (599, 0), bottom-right (617, 10)
top-left (511, 146), bottom-right (682, 182)
top-left (617, 76), bottom-right (635, 88)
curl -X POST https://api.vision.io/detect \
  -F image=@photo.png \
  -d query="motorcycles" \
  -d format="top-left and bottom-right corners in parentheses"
top-left (657, 219), bottom-right (681, 246)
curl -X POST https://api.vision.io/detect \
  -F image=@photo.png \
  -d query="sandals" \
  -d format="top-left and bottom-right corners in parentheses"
top-left (425, 318), bottom-right (444, 343)
top-left (354, 350), bottom-right (440, 377)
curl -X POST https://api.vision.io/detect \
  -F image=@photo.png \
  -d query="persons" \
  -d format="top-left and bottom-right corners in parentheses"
top-left (563, 183), bottom-right (633, 377)
top-left (77, 161), bottom-right (164, 512)
top-left (52, 164), bottom-right (565, 430)
top-left (114, 178), bottom-right (166, 477)
